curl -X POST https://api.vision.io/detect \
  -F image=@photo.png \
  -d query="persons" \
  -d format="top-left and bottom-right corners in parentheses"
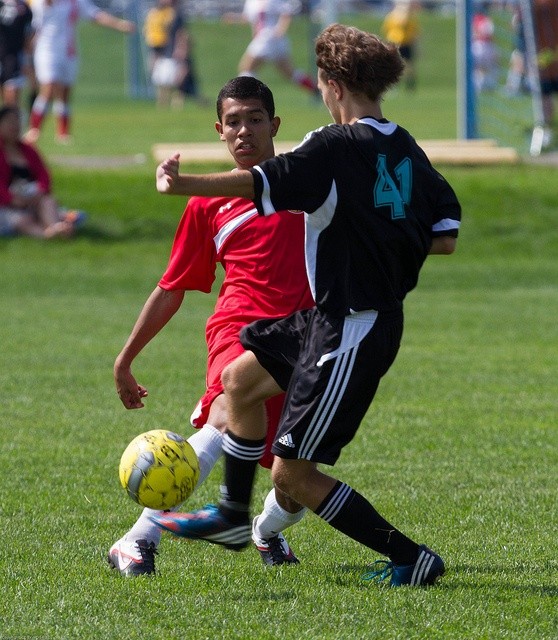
top-left (233, 2), bottom-right (319, 94)
top-left (24, 1), bottom-right (135, 142)
top-left (0, 106), bottom-right (85, 241)
top-left (310, 0), bottom-right (339, 68)
top-left (148, 22), bottom-right (462, 591)
top-left (1, 2), bottom-right (36, 107)
top-left (142, 1), bottom-right (196, 106)
top-left (501, 13), bottom-right (525, 99)
top-left (108, 79), bottom-right (307, 580)
top-left (467, 17), bottom-right (498, 100)
top-left (377, 2), bottom-right (419, 89)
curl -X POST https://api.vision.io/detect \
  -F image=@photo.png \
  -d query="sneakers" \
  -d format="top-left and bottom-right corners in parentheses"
top-left (361, 544), bottom-right (445, 588)
top-left (107, 532), bottom-right (160, 578)
top-left (250, 515), bottom-right (301, 568)
top-left (146, 504), bottom-right (252, 551)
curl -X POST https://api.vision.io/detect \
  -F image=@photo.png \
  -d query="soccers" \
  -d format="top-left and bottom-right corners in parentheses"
top-left (116, 428), bottom-right (202, 511)
